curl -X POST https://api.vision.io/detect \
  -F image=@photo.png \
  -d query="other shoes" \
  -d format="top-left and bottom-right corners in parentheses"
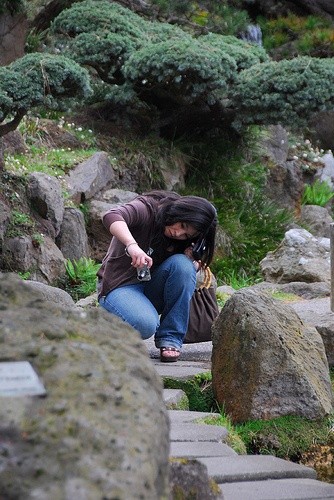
top-left (160, 347), bottom-right (180, 361)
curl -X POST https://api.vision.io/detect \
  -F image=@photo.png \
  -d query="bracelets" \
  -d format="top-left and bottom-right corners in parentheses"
top-left (124, 242), bottom-right (139, 258)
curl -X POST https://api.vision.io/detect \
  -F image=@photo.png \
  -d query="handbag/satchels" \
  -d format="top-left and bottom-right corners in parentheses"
top-left (181, 260), bottom-right (221, 344)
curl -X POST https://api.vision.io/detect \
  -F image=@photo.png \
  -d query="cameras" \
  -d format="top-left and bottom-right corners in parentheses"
top-left (136, 263), bottom-right (151, 282)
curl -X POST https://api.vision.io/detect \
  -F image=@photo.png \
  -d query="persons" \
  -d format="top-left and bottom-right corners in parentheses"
top-left (95, 190), bottom-right (218, 362)
